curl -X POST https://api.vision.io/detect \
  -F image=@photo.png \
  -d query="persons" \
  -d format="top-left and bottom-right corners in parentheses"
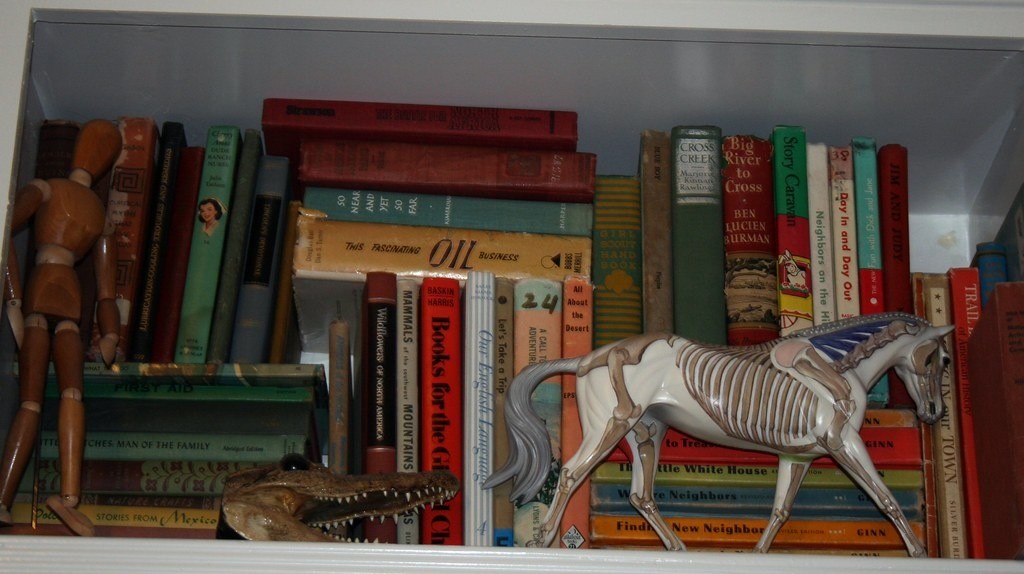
top-left (5, 119), bottom-right (122, 536)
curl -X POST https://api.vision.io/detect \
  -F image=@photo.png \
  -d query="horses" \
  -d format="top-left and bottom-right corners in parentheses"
top-left (480, 311), bottom-right (955, 558)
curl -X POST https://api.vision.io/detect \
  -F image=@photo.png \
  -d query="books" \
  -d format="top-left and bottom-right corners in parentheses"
top-left (1, 94), bottom-right (1024, 560)
